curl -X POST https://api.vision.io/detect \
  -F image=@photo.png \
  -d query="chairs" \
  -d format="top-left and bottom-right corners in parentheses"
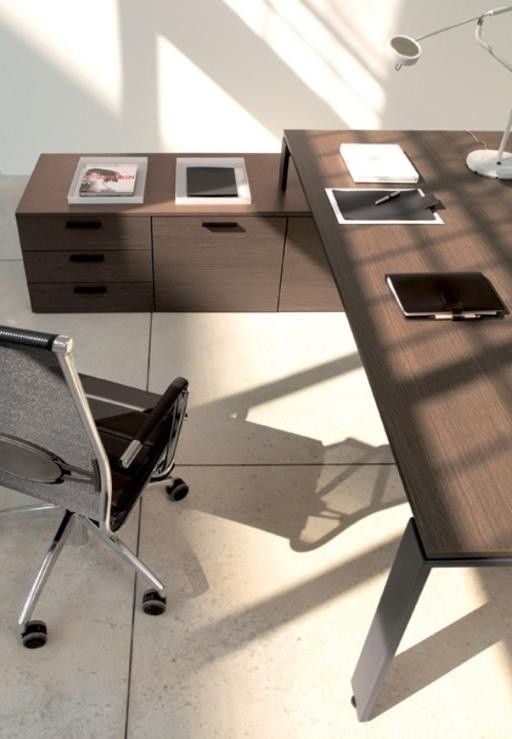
top-left (1, 324), bottom-right (191, 649)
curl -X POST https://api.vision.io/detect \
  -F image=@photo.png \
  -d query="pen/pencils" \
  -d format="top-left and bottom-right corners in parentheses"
top-left (375, 191), bottom-right (400, 205)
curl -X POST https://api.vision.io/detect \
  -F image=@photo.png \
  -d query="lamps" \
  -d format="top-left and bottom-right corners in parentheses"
top-left (388, 9), bottom-right (512, 185)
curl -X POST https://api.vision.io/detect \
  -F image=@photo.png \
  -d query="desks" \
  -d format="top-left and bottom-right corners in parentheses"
top-left (278, 129), bottom-right (511, 721)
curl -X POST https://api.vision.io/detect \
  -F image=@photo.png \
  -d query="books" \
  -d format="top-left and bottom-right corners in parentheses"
top-left (384, 269), bottom-right (507, 322)
top-left (79, 162), bottom-right (140, 199)
